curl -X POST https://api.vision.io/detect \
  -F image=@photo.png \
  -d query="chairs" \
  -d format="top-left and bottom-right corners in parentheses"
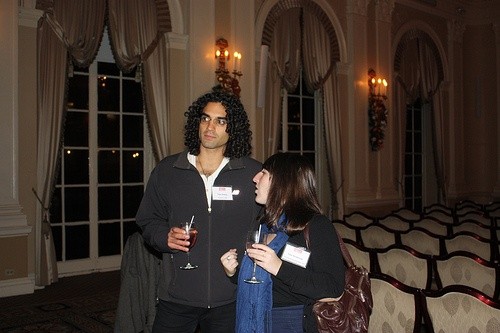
top-left (330, 198), bottom-right (500, 333)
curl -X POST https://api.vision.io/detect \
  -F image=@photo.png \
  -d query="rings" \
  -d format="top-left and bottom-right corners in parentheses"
top-left (227, 257), bottom-right (231, 260)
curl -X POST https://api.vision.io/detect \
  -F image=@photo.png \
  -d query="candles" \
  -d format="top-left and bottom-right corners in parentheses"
top-left (215, 50), bottom-right (242, 74)
top-left (370, 77), bottom-right (388, 98)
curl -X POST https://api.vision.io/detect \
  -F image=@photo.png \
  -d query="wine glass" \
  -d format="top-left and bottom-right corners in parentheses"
top-left (243, 223), bottom-right (269, 284)
top-left (178, 222), bottom-right (199, 270)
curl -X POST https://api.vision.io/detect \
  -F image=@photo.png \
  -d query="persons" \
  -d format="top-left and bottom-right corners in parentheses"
top-left (135, 92), bottom-right (264, 333)
top-left (220, 151), bottom-right (345, 333)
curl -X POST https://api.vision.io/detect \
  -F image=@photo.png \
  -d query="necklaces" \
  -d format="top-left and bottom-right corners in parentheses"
top-left (198, 157), bottom-right (211, 177)
